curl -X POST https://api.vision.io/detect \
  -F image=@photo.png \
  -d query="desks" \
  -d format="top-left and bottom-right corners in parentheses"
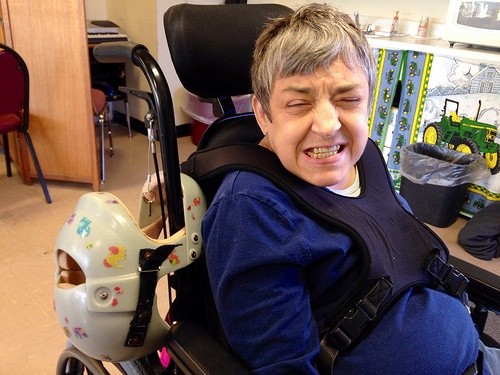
top-left (366, 33), bottom-right (500, 218)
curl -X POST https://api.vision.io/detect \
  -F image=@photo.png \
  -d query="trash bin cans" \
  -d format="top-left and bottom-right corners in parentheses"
top-left (399, 142), bottom-right (487, 228)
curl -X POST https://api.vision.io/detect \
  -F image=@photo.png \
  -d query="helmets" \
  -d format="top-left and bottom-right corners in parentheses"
top-left (53, 169), bottom-right (206, 362)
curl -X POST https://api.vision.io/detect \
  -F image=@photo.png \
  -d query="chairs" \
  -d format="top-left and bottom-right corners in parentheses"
top-left (90, 88), bottom-right (114, 185)
top-left (0, 42), bottom-right (51, 203)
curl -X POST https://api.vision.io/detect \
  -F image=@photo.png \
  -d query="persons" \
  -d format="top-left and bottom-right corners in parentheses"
top-left (201, 3), bottom-right (500, 375)
